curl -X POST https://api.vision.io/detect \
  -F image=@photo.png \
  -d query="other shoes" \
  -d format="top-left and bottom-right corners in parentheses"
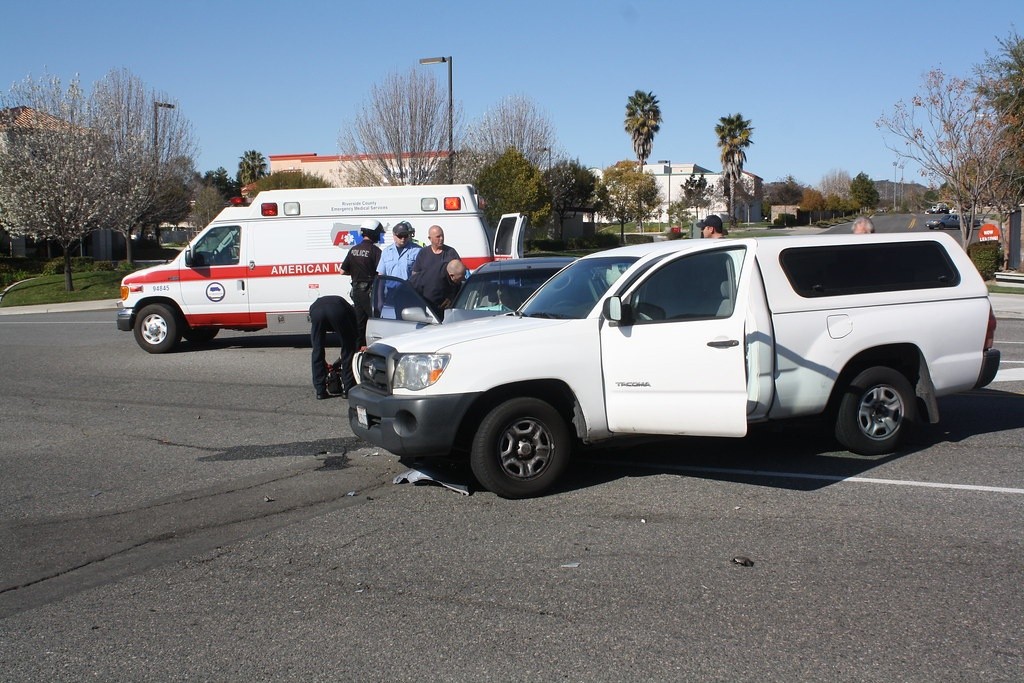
top-left (342, 389), bottom-right (349, 398)
top-left (317, 390), bottom-right (328, 400)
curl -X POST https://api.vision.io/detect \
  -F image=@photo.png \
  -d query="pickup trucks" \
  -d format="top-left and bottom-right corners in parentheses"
top-left (925, 215), bottom-right (980, 231)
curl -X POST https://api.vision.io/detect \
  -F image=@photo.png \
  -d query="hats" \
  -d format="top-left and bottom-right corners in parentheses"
top-left (696, 214), bottom-right (723, 229)
top-left (394, 224), bottom-right (410, 236)
top-left (402, 221), bottom-right (415, 232)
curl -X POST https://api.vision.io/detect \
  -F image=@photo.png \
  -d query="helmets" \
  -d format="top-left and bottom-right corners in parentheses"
top-left (360, 220), bottom-right (386, 235)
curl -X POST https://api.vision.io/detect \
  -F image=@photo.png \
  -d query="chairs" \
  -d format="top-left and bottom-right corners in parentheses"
top-left (688, 266), bottom-right (732, 315)
top-left (643, 277), bottom-right (681, 317)
top-left (480, 284), bottom-right (521, 310)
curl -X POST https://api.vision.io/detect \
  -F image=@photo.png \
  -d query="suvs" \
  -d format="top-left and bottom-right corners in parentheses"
top-left (347, 231), bottom-right (1001, 499)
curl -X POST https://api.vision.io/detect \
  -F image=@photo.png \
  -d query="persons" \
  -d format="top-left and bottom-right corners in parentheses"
top-left (851, 217), bottom-right (874, 233)
top-left (309, 296), bottom-right (359, 400)
top-left (340, 219), bottom-right (385, 349)
top-left (400, 221), bottom-right (426, 248)
top-left (386, 260), bottom-right (465, 316)
top-left (376, 224), bottom-right (422, 311)
top-left (411, 225), bottom-right (460, 275)
top-left (696, 214), bottom-right (723, 238)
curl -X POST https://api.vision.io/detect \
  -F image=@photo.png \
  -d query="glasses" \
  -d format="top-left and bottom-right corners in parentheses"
top-left (410, 233), bottom-right (415, 237)
top-left (395, 234), bottom-right (408, 239)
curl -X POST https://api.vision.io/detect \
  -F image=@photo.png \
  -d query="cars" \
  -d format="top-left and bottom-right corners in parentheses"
top-left (925, 203), bottom-right (957, 214)
top-left (365, 256), bottom-right (608, 347)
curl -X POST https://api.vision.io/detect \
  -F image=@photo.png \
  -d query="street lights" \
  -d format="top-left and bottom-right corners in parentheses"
top-left (538, 145), bottom-right (551, 169)
top-left (419, 56), bottom-right (454, 184)
top-left (154, 102), bottom-right (174, 175)
top-left (657, 160), bottom-right (671, 225)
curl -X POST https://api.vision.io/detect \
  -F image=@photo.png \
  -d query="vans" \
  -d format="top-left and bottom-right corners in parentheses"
top-left (116, 182), bottom-right (528, 353)
